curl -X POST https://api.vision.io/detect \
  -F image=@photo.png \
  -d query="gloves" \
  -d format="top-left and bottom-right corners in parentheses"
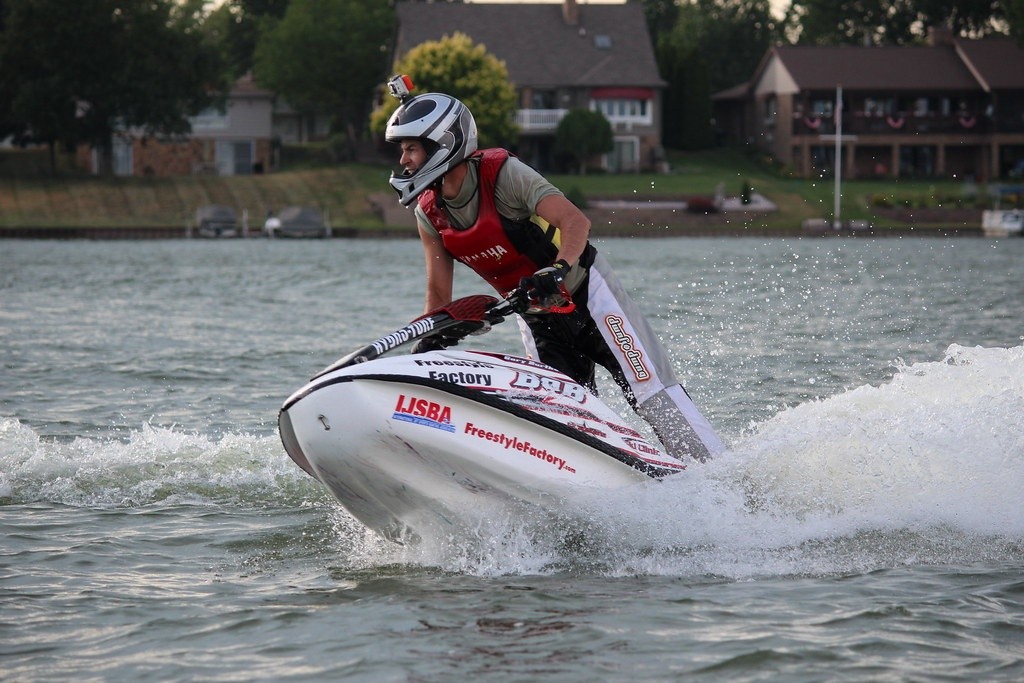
top-left (527, 258), bottom-right (572, 302)
top-left (410, 338), bottom-right (445, 354)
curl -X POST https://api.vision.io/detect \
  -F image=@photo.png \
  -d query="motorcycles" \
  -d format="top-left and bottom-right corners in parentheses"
top-left (277, 275), bottom-right (698, 559)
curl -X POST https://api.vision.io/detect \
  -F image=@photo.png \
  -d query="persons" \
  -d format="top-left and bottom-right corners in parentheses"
top-left (383, 91), bottom-right (729, 463)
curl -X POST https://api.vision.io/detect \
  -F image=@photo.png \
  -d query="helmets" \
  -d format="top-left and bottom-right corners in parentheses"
top-left (384, 92), bottom-right (477, 206)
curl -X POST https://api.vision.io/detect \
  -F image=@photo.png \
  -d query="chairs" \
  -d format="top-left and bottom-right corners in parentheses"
top-left (179, 203), bottom-right (250, 238)
top-left (267, 201), bottom-right (333, 238)
